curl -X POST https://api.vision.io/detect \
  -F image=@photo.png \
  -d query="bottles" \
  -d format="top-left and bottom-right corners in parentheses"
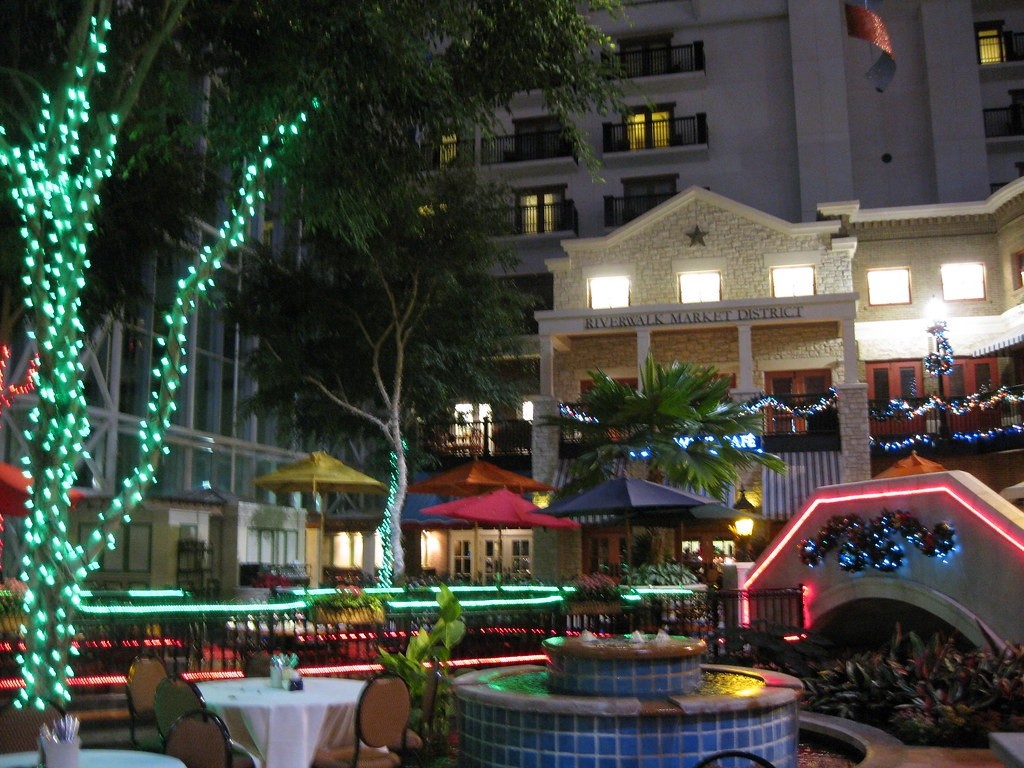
top-left (270, 650), bottom-right (283, 689)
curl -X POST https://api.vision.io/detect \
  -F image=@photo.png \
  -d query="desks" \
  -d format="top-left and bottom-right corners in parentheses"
top-left (195, 676), bottom-right (389, 768)
top-left (0, 748), bottom-right (187, 768)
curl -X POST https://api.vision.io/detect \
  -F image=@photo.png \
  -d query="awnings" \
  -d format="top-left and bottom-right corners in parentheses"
top-left (762, 451), bottom-right (840, 520)
top-left (401, 471), bottom-right (473, 524)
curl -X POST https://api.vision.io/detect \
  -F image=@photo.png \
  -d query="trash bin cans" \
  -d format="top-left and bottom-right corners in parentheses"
top-left (721, 562), bottom-right (754, 627)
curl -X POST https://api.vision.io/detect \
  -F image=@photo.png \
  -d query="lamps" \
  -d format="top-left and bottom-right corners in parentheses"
top-left (732, 486), bottom-right (755, 553)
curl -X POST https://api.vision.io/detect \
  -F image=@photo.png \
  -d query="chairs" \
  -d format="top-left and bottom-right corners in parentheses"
top-left (389, 655), bottom-right (441, 768)
top-left (154, 676), bottom-right (208, 754)
top-left (163, 709), bottom-right (232, 768)
top-left (126, 653), bottom-right (166, 751)
top-left (315, 671), bottom-right (413, 768)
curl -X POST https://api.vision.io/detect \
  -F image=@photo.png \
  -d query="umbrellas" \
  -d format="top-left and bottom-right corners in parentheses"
top-left (407, 452), bottom-right (559, 585)
top-left (600, 502), bottom-right (767, 583)
top-left (873, 449), bottom-right (946, 478)
top-left (528, 468), bottom-right (724, 632)
top-left (419, 483), bottom-right (581, 585)
top-left (254, 451), bottom-right (389, 588)
